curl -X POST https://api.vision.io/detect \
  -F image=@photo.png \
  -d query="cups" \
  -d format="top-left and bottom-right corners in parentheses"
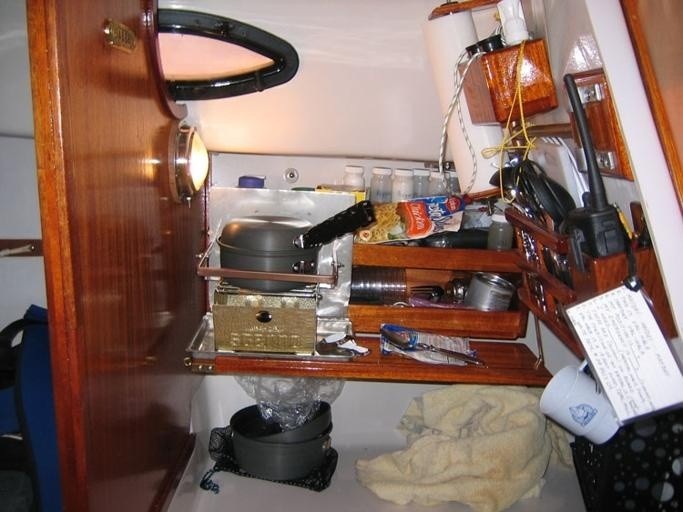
top-left (351, 266), bottom-right (409, 303)
top-left (539, 358), bottom-right (620, 445)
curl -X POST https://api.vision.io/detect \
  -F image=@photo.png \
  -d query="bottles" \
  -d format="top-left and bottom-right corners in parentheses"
top-left (449, 170), bottom-right (461, 195)
top-left (487, 214), bottom-right (514, 250)
top-left (431, 170), bottom-right (449, 197)
top-left (370, 167), bottom-right (392, 206)
top-left (342, 166), bottom-right (365, 192)
top-left (393, 170), bottom-right (414, 204)
top-left (414, 169), bottom-right (430, 197)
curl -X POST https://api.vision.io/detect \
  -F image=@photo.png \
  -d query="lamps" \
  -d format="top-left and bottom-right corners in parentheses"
top-left (169, 119), bottom-right (210, 204)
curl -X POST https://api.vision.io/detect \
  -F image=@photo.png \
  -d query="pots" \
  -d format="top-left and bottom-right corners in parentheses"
top-left (229, 401), bottom-right (333, 480)
top-left (216, 200), bottom-right (377, 291)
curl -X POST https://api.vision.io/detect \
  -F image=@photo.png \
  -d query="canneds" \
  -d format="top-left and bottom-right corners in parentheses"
top-left (465, 271), bottom-right (514, 312)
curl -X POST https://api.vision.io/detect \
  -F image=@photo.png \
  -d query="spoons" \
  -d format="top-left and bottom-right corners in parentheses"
top-left (528, 173), bottom-right (576, 286)
top-left (319, 332), bottom-right (371, 357)
top-left (489, 167), bottom-right (518, 190)
top-left (378, 326), bottom-right (487, 368)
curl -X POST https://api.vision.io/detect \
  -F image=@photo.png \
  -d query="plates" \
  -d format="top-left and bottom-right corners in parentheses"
top-left (185, 310), bottom-right (356, 359)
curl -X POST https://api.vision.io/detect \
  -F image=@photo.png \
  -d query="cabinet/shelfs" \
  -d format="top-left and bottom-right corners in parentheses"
top-left (183, 176), bottom-right (556, 387)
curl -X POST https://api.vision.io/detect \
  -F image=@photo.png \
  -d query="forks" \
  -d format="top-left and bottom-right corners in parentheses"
top-left (412, 283), bottom-right (445, 297)
